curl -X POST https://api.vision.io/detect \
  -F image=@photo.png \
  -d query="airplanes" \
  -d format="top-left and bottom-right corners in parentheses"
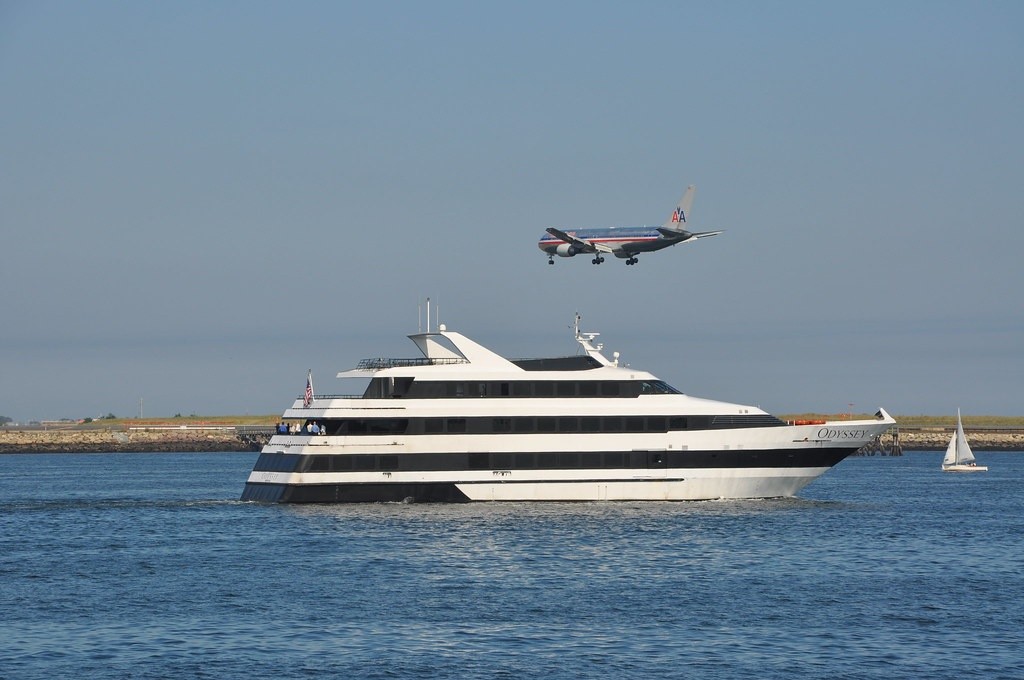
top-left (537, 182), bottom-right (727, 267)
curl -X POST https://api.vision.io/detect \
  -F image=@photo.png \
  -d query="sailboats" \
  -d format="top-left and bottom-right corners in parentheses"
top-left (940, 408), bottom-right (990, 472)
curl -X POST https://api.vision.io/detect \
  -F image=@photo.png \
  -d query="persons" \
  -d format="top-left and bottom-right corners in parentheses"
top-left (307, 421), bottom-right (326, 435)
top-left (276, 421), bottom-right (301, 435)
top-left (970, 460), bottom-right (977, 466)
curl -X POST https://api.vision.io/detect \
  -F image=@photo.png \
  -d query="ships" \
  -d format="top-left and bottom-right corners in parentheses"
top-left (238, 293), bottom-right (897, 504)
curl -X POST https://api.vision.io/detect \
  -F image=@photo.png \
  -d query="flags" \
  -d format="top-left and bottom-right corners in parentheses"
top-left (303, 378), bottom-right (311, 407)
